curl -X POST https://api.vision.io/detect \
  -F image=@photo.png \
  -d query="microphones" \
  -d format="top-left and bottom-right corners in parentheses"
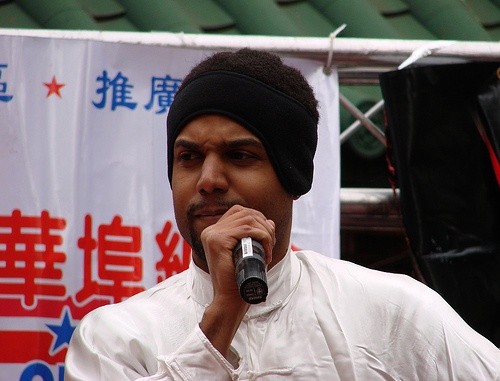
top-left (233, 236), bottom-right (268, 304)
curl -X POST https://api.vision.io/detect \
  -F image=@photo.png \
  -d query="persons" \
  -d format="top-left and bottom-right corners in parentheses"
top-left (62, 49), bottom-right (500, 381)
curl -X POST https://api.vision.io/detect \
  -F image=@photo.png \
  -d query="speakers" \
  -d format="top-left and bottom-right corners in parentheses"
top-left (382, 58), bottom-right (500, 291)
